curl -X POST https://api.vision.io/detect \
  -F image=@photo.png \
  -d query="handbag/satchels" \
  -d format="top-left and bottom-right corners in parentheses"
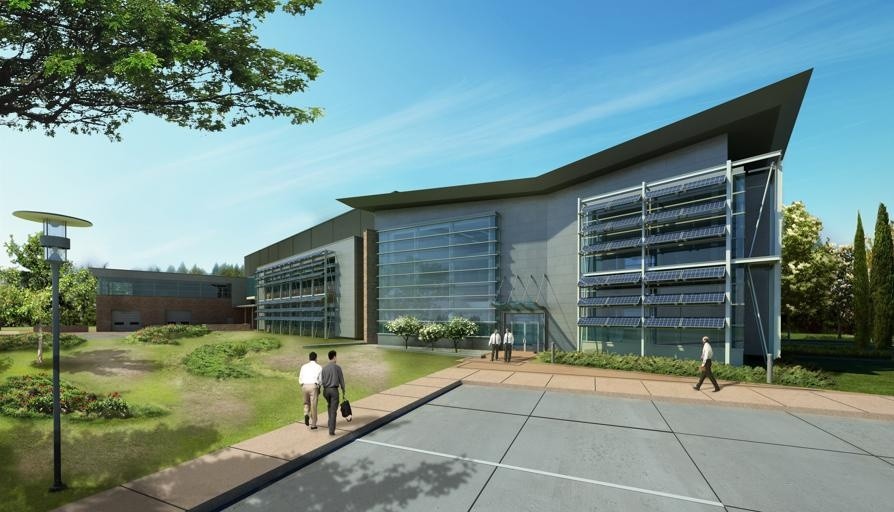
top-left (341, 400), bottom-right (352, 417)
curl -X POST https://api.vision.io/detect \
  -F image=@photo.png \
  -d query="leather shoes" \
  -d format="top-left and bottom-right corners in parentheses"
top-left (305, 415), bottom-right (309, 426)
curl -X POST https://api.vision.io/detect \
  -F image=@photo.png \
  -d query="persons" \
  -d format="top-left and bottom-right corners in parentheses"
top-left (488, 328), bottom-right (503, 361)
top-left (502, 329), bottom-right (514, 362)
top-left (321, 349), bottom-right (346, 435)
top-left (692, 336), bottom-right (721, 393)
top-left (298, 351), bottom-right (323, 430)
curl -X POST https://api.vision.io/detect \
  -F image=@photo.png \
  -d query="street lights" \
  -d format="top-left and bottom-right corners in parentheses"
top-left (11, 209), bottom-right (94, 493)
